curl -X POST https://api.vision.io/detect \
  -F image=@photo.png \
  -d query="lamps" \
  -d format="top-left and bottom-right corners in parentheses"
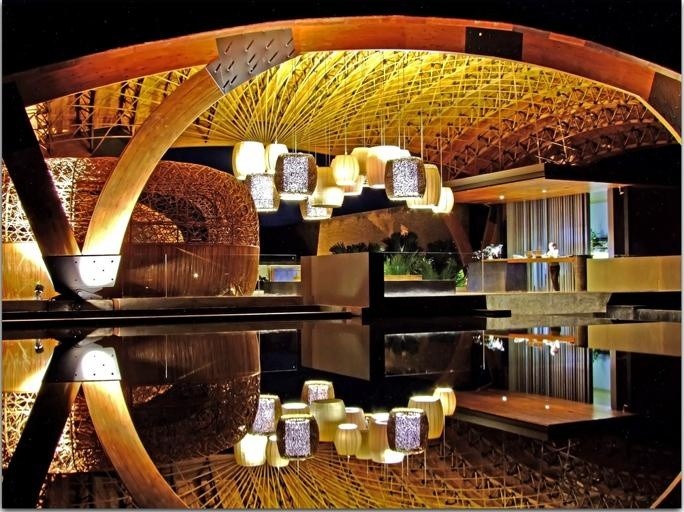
top-left (231, 50), bottom-right (455, 222)
top-left (34, 339), bottom-right (43, 354)
top-left (235, 380), bottom-right (457, 510)
top-left (34, 283), bottom-right (44, 300)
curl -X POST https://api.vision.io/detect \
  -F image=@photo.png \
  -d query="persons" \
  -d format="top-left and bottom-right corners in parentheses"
top-left (547, 242), bottom-right (558, 258)
top-left (545, 339), bottom-right (560, 355)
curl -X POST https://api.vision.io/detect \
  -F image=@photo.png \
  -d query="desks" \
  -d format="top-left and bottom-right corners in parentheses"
top-left (449, 390), bottom-right (633, 494)
top-left (509, 326), bottom-right (587, 347)
top-left (587, 321), bottom-right (681, 357)
top-left (587, 255), bottom-right (682, 291)
top-left (507, 254), bottom-right (587, 291)
